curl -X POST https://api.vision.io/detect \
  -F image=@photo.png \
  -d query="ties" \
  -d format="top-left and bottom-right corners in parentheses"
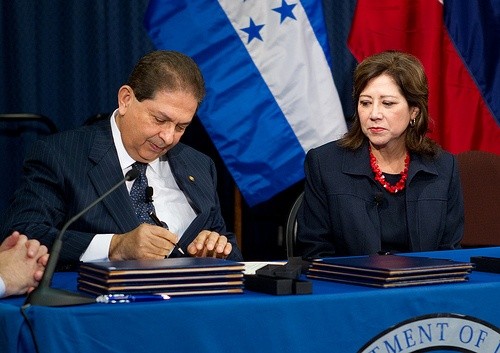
top-left (129, 161), bottom-right (156, 225)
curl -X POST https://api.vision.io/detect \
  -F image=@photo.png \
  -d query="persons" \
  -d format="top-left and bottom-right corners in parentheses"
top-left (0, 231), bottom-right (49, 299)
top-left (295, 50), bottom-right (464, 256)
top-left (7, 50), bottom-right (241, 269)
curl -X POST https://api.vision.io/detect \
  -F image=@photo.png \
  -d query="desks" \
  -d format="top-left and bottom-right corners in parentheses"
top-left (0, 247), bottom-right (500, 353)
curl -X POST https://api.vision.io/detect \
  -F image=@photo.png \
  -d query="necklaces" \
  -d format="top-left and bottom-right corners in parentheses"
top-left (369, 152), bottom-right (411, 193)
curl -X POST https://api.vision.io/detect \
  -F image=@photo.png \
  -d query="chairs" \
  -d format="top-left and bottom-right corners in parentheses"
top-left (457, 151), bottom-right (500, 246)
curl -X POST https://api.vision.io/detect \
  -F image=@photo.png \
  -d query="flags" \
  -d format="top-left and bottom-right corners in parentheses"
top-left (335, 0), bottom-right (500, 156)
top-left (147, 0), bottom-right (350, 208)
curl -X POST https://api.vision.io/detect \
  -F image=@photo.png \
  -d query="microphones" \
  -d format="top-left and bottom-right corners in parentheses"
top-left (24, 169), bottom-right (140, 307)
top-left (145, 187), bottom-right (154, 202)
top-left (375, 192), bottom-right (383, 200)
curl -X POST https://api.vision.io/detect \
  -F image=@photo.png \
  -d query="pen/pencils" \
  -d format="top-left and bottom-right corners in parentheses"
top-left (96, 293), bottom-right (172, 304)
top-left (147, 210), bottom-right (185, 254)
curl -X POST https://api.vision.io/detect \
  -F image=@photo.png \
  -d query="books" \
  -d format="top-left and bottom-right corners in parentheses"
top-left (240, 261), bottom-right (288, 274)
top-left (78, 256), bottom-right (244, 300)
top-left (307, 256), bottom-right (474, 287)
